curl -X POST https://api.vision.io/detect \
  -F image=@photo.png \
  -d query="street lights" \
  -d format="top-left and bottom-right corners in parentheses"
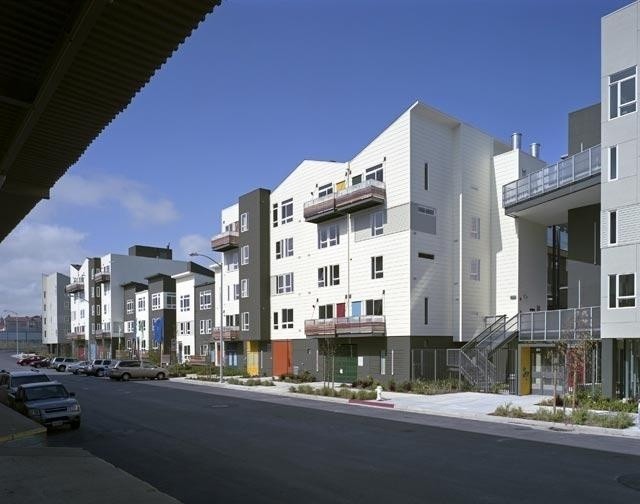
top-left (188, 252), bottom-right (225, 384)
top-left (3, 309), bottom-right (19, 355)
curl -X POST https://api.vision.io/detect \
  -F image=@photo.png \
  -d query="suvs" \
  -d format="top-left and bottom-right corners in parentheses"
top-left (86, 359), bottom-right (116, 377)
top-left (0, 367), bottom-right (52, 409)
top-left (52, 357), bottom-right (78, 372)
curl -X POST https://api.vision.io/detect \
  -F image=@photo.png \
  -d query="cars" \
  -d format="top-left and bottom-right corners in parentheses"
top-left (15, 380), bottom-right (82, 435)
top-left (108, 360), bottom-right (169, 381)
top-left (67, 360), bottom-right (90, 375)
top-left (16, 355), bottom-right (51, 367)
